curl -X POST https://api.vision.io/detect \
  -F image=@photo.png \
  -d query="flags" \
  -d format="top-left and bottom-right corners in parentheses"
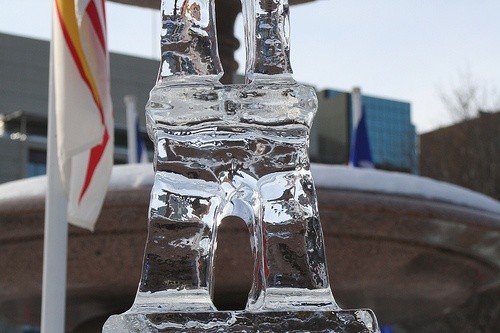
top-left (348, 101), bottom-right (378, 169)
top-left (52, 0), bottom-right (116, 234)
